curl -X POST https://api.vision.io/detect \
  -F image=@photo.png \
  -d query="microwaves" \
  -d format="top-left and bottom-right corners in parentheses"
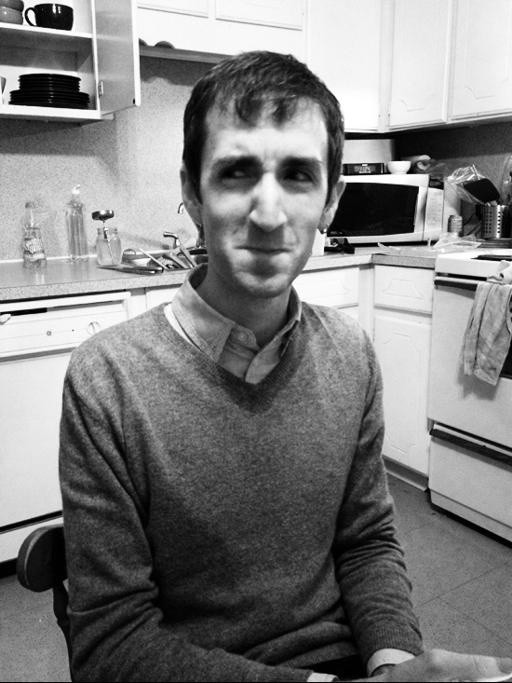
top-left (325, 173), bottom-right (446, 252)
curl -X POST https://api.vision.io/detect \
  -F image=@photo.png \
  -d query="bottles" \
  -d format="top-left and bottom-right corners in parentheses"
top-left (96, 225), bottom-right (124, 268)
top-left (21, 200), bottom-right (49, 272)
top-left (63, 183), bottom-right (91, 265)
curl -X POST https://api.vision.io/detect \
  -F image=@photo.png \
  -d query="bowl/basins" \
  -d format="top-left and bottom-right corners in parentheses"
top-left (386, 158), bottom-right (413, 175)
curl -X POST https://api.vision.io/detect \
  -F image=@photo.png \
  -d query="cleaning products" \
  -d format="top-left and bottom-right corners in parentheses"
top-left (65, 184), bottom-right (87, 258)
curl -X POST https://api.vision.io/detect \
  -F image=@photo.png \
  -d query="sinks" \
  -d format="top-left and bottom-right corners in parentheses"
top-left (98, 253), bottom-right (207, 274)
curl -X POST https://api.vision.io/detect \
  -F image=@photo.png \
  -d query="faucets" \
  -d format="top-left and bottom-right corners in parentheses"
top-left (177, 202), bottom-right (185, 215)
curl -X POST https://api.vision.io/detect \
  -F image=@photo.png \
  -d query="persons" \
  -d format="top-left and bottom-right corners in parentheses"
top-left (58, 49), bottom-right (511, 681)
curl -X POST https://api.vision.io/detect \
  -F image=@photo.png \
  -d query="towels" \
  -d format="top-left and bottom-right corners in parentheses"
top-left (459, 273), bottom-right (512, 389)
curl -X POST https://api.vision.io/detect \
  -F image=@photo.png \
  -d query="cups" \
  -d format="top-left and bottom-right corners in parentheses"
top-left (25, 2), bottom-right (74, 32)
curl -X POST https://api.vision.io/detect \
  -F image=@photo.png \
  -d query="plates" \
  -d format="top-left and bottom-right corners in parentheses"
top-left (7, 72), bottom-right (90, 110)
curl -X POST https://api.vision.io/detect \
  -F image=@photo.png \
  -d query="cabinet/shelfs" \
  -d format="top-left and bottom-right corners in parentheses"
top-left (1, 0), bottom-right (140, 125)
top-left (366, 267), bottom-right (431, 496)
top-left (291, 262), bottom-right (370, 326)
top-left (304, 1), bottom-right (511, 133)
top-left (139, 1), bottom-right (304, 67)
